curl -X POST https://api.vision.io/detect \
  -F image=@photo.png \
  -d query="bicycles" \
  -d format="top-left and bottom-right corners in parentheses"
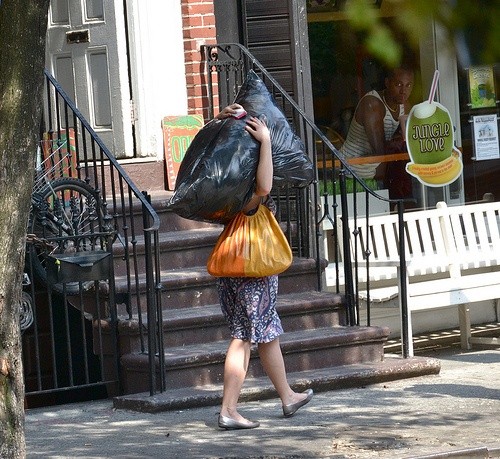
top-left (17, 139), bottom-right (107, 297)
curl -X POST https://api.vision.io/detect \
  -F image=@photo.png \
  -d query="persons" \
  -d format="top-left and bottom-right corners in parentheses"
top-left (214, 104), bottom-right (313, 431)
top-left (340, 66), bottom-right (416, 179)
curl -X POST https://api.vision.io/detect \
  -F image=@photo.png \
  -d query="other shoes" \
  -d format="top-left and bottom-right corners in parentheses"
top-left (282, 388), bottom-right (313, 417)
top-left (217, 414), bottom-right (260, 430)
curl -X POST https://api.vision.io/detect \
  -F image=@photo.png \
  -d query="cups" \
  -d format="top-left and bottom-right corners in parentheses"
top-left (400, 115), bottom-right (410, 141)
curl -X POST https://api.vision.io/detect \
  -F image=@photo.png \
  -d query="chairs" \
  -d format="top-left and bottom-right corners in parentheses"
top-left (313, 124), bottom-right (346, 161)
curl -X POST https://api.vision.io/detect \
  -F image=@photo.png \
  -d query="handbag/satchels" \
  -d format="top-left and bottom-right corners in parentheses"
top-left (206, 196), bottom-right (293, 278)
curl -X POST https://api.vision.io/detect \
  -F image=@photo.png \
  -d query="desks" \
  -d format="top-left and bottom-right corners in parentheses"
top-left (315, 153), bottom-right (411, 169)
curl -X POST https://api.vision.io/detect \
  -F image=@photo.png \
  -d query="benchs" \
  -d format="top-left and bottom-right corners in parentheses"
top-left (323, 201), bottom-right (500, 357)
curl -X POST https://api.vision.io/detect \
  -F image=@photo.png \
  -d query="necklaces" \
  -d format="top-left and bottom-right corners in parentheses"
top-left (383, 88), bottom-right (399, 113)
